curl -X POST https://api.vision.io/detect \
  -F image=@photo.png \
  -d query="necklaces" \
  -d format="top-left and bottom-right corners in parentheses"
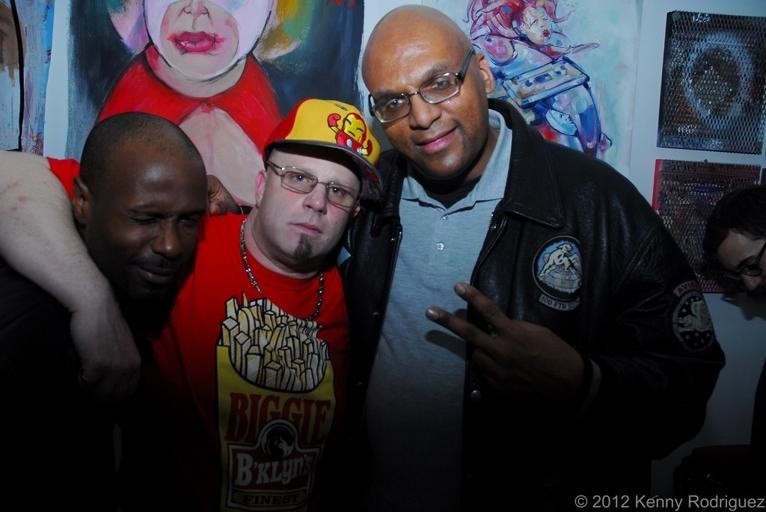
top-left (237, 214), bottom-right (326, 325)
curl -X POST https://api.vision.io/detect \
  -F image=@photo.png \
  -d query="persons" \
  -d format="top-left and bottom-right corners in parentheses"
top-left (0, 97), bottom-right (383, 507)
top-left (704, 183), bottom-right (765, 510)
top-left (514, 7), bottom-right (557, 45)
top-left (203, 4), bottom-right (721, 507)
top-left (0, 110), bottom-right (208, 507)
top-left (84, 0), bottom-right (291, 202)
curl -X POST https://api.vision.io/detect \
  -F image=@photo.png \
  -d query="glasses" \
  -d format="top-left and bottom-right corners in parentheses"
top-left (720, 242), bottom-right (766, 288)
top-left (268, 161), bottom-right (360, 217)
top-left (367, 51), bottom-right (474, 125)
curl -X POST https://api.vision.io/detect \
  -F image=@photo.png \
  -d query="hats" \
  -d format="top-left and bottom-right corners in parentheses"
top-left (262, 98), bottom-right (386, 201)
